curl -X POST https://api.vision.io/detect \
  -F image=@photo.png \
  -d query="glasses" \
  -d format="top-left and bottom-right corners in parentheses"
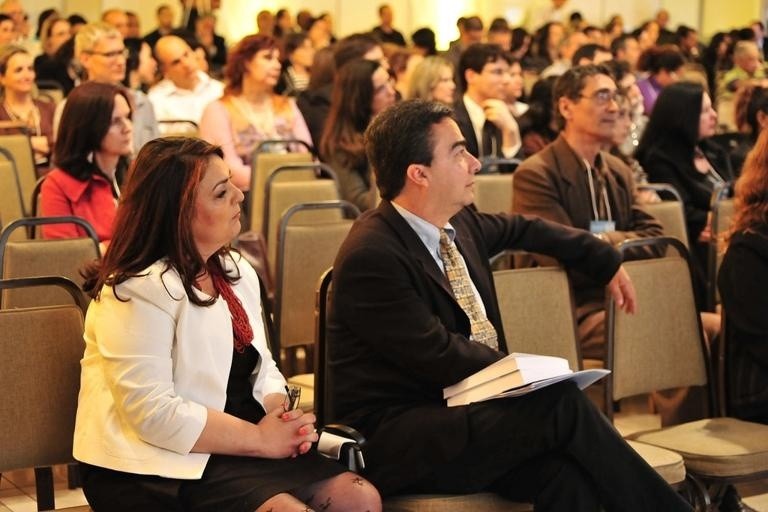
top-left (582, 92), bottom-right (622, 106)
top-left (283, 384), bottom-right (301, 411)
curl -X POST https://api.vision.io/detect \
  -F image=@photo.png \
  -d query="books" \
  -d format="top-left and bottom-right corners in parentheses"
top-left (441, 351), bottom-right (614, 410)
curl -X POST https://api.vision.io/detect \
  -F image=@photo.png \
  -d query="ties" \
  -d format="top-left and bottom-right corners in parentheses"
top-left (483, 124), bottom-right (492, 157)
top-left (440, 229), bottom-right (499, 354)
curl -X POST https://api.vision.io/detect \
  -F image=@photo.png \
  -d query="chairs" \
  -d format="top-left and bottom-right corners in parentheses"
top-left (0, 80), bottom-right (768, 511)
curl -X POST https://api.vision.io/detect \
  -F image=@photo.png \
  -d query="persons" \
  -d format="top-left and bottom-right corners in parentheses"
top-left (258, 9), bottom-right (339, 98)
top-left (634, 83), bottom-right (736, 340)
top-left (609, 8), bottom-right (768, 132)
top-left (200, 34), bottom-right (321, 234)
top-left (372, 4), bottom-right (408, 46)
top-left (40, 81), bottom-right (135, 256)
top-left (712, 126), bottom-right (767, 427)
top-left (720, 81), bottom-right (767, 199)
top-left (70, 135), bottom-right (388, 512)
top-left (321, 98), bottom-right (702, 512)
top-left (506, 61), bottom-right (758, 426)
top-left (0, 6), bottom-right (228, 154)
top-left (297, 11), bottom-right (625, 222)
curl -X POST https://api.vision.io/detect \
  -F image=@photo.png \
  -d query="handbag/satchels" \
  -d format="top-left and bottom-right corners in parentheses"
top-left (313, 428), bottom-right (367, 476)
